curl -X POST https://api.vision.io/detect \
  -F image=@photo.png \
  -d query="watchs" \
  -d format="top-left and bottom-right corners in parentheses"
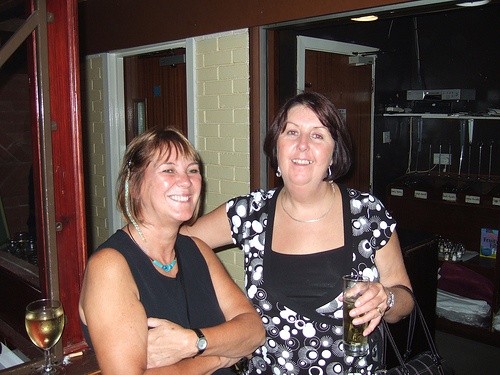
top-left (191, 328), bottom-right (207, 359)
top-left (386, 287), bottom-right (394, 312)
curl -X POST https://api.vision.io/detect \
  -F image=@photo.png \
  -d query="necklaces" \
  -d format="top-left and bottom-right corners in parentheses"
top-left (127, 223), bottom-right (177, 272)
top-left (281, 181), bottom-right (335, 223)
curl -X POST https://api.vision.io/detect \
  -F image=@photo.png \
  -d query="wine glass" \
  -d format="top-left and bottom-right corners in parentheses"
top-left (24, 298), bottom-right (67, 375)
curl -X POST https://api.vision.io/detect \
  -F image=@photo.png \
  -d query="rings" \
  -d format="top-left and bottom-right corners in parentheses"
top-left (375, 307), bottom-right (383, 314)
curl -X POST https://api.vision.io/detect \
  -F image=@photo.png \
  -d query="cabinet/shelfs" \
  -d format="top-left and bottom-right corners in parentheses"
top-left (385, 171), bottom-right (500, 348)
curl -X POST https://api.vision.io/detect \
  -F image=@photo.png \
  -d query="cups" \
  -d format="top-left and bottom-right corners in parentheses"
top-left (342, 275), bottom-right (370, 356)
top-left (8, 232), bottom-right (38, 264)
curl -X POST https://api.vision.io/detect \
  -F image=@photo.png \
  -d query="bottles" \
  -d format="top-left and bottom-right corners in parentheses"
top-left (437, 235), bottom-right (466, 262)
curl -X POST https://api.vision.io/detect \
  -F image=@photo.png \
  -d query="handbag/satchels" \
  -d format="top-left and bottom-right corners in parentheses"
top-left (375, 285), bottom-right (449, 375)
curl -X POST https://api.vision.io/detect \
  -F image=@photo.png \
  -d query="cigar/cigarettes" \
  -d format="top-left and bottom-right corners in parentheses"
top-left (68, 351), bottom-right (83, 358)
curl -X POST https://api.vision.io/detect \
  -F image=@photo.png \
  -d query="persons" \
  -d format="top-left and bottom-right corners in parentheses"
top-left (179, 91), bottom-right (414, 375)
top-left (78, 126), bottom-right (265, 375)
